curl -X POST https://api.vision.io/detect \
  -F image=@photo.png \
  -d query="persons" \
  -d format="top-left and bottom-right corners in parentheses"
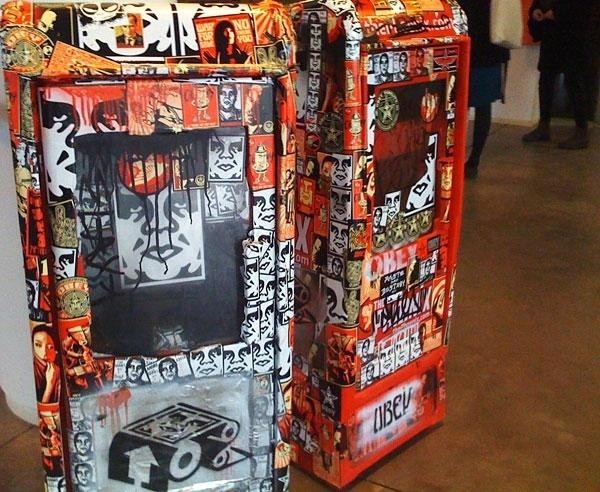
top-left (458, 0), bottom-right (510, 179)
top-left (521, 1), bottom-right (599, 149)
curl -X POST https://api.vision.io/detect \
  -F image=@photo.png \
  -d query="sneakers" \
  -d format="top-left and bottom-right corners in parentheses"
top-left (559, 131), bottom-right (590, 149)
top-left (522, 124), bottom-right (552, 143)
top-left (464, 159), bottom-right (479, 179)
